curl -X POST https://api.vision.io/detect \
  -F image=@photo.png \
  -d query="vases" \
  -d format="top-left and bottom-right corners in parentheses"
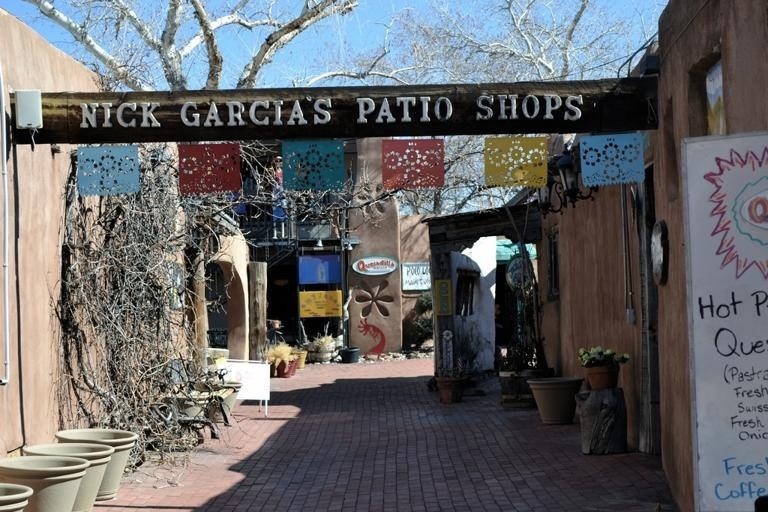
top-left (586, 366), bottom-right (620, 390)
top-left (0, 427), bottom-right (140, 512)
top-left (527, 374), bottom-right (585, 425)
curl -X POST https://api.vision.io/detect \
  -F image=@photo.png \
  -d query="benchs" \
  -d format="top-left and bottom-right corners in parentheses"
top-left (153, 356), bottom-right (236, 441)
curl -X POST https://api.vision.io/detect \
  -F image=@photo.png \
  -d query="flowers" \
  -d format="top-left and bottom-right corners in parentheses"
top-left (575, 344), bottom-right (632, 369)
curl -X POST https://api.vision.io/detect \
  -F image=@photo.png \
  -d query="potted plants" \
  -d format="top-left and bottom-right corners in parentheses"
top-left (260, 340), bottom-right (308, 378)
top-left (436, 367), bottom-right (472, 404)
